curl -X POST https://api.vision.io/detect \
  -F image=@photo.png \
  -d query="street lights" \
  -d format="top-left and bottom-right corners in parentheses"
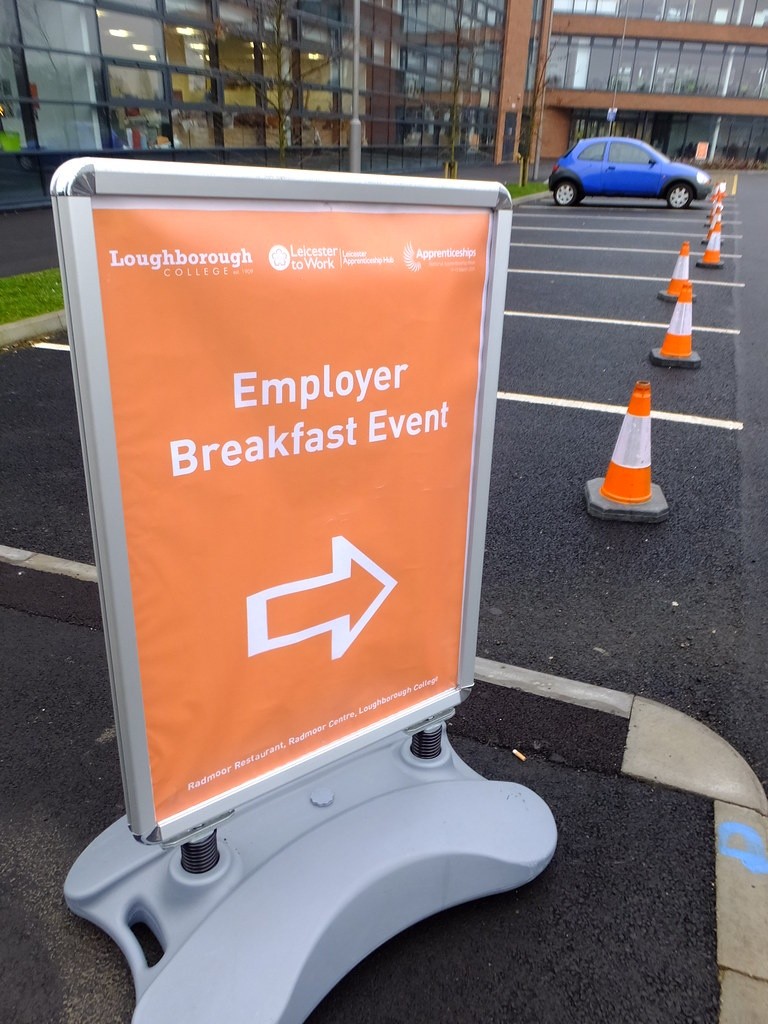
top-left (709, 179), bottom-right (728, 202)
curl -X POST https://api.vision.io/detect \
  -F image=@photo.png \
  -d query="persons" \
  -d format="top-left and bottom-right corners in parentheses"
top-left (310, 125), bottom-right (323, 146)
top-left (670, 140), bottom-right (768, 166)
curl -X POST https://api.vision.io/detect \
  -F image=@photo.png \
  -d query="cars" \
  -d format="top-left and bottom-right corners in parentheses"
top-left (19, 120), bottom-right (129, 174)
top-left (548, 136), bottom-right (712, 209)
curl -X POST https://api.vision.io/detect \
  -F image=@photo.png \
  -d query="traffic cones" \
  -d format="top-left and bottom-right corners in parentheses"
top-left (650, 283), bottom-right (702, 368)
top-left (704, 195), bottom-right (724, 227)
top-left (700, 205), bottom-right (724, 245)
top-left (583, 380), bottom-right (670, 523)
top-left (696, 222), bottom-right (724, 268)
top-left (658, 241), bottom-right (697, 303)
top-left (705, 189), bottom-right (725, 218)
